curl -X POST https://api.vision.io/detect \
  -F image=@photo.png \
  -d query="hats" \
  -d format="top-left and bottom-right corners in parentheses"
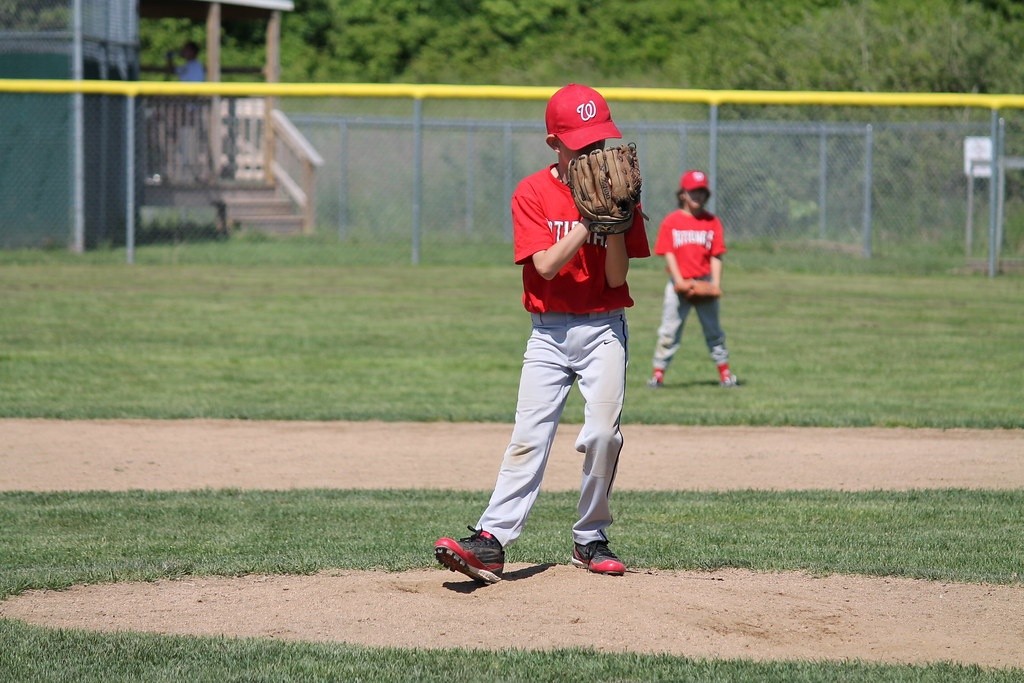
top-left (681, 170), bottom-right (709, 190)
top-left (546, 83), bottom-right (622, 152)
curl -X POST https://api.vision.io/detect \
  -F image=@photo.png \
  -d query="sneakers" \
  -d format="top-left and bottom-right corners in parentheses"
top-left (572, 530), bottom-right (626, 574)
top-left (434, 525), bottom-right (505, 586)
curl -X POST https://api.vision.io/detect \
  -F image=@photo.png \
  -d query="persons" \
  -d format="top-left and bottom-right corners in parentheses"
top-left (647, 170), bottom-right (740, 387)
top-left (434, 83), bottom-right (652, 585)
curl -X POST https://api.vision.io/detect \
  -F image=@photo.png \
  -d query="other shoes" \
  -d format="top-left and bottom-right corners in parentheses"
top-left (648, 368), bottom-right (665, 387)
top-left (717, 363), bottom-right (738, 387)
